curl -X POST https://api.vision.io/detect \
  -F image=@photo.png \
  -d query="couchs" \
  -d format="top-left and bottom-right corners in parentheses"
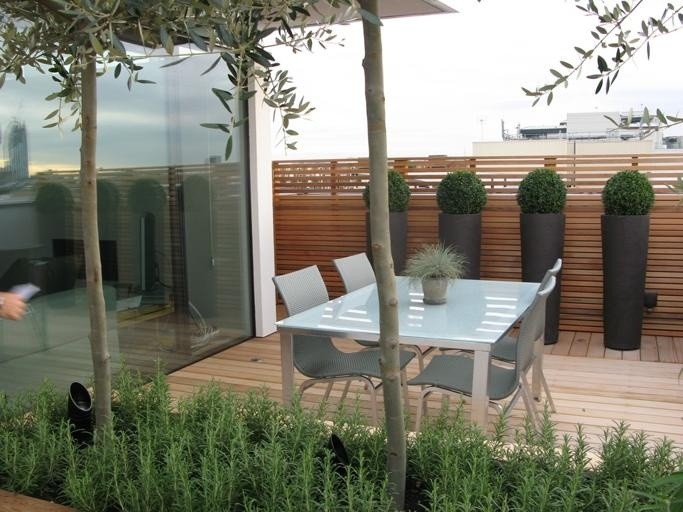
top-left (1, 284), bottom-right (121, 413)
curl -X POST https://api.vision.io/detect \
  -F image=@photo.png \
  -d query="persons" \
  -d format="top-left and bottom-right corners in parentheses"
top-left (0, 292), bottom-right (27, 322)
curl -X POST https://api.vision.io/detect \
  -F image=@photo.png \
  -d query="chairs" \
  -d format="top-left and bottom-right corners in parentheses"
top-left (270, 251), bottom-right (563, 443)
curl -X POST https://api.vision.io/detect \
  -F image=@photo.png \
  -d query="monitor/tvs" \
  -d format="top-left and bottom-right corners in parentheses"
top-left (127, 211), bottom-right (169, 307)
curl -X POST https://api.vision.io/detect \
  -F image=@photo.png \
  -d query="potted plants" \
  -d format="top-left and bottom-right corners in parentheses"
top-left (365, 171), bottom-right (412, 276)
top-left (517, 169), bottom-right (568, 346)
top-left (404, 238), bottom-right (469, 304)
top-left (600, 168), bottom-right (653, 351)
top-left (437, 171), bottom-right (488, 280)
